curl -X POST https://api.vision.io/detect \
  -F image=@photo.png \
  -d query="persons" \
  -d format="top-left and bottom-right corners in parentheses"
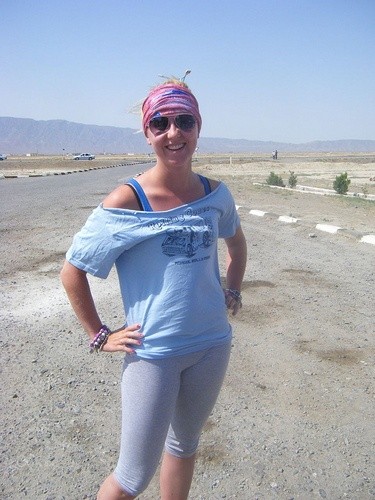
top-left (272, 150), bottom-right (279, 159)
top-left (59, 76), bottom-right (247, 500)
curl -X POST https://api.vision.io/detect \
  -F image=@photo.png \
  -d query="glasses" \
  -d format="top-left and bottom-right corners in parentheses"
top-left (147, 114), bottom-right (197, 136)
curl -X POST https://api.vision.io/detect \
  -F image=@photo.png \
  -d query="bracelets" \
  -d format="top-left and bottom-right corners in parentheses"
top-left (89, 324), bottom-right (112, 352)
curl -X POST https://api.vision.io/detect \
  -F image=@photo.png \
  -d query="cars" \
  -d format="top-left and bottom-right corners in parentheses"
top-left (72, 153), bottom-right (96, 160)
top-left (0, 154), bottom-right (7, 161)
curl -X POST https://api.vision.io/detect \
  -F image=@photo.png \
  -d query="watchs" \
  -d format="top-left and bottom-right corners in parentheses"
top-left (225, 287), bottom-right (242, 300)
